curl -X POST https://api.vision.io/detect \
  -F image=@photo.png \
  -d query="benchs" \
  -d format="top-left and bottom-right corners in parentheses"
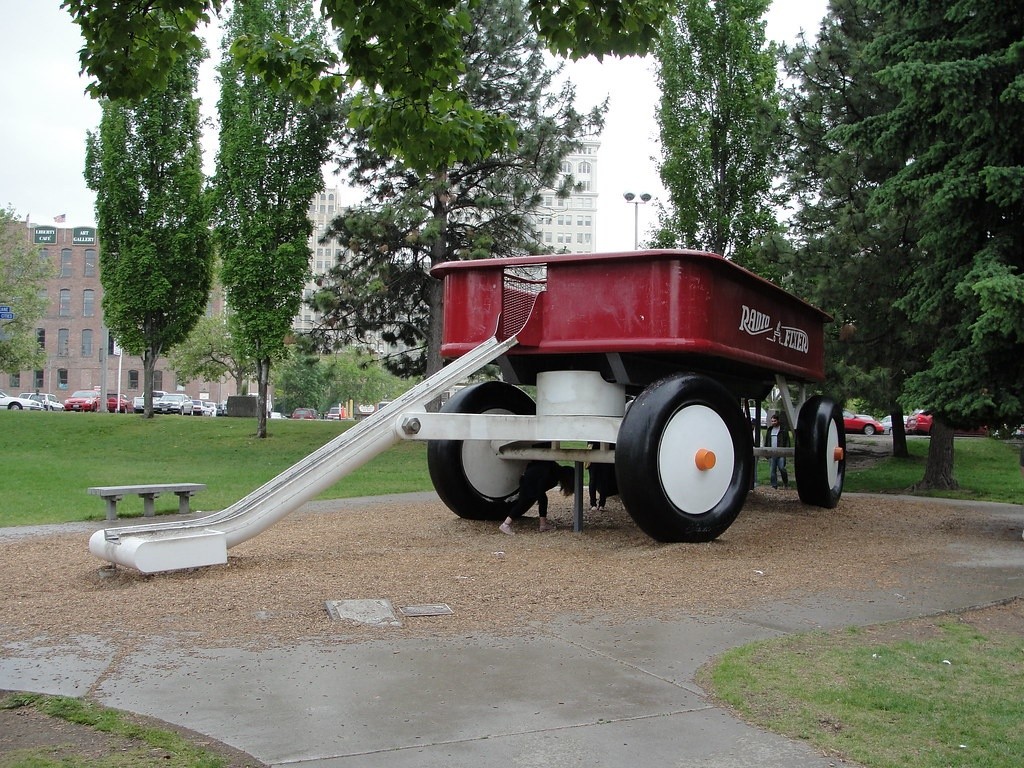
top-left (87, 483), bottom-right (206, 519)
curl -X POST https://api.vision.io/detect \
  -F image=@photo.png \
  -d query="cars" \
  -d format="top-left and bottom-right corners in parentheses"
top-left (203, 402), bottom-right (228, 417)
top-left (106, 393), bottom-right (134, 414)
top-left (310, 409), bottom-right (321, 419)
top-left (907, 409), bottom-right (996, 437)
top-left (153, 394), bottom-right (194, 416)
top-left (843, 409), bottom-right (886, 436)
top-left (855, 415), bottom-right (873, 420)
top-left (292, 407), bottom-right (315, 419)
top-left (18, 393), bottom-right (65, 412)
top-left (192, 401), bottom-right (206, 416)
top-left (880, 415), bottom-right (910, 436)
top-left (0, 390), bottom-right (43, 411)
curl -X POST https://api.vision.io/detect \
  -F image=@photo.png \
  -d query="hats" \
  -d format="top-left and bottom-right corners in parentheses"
top-left (750, 418), bottom-right (756, 424)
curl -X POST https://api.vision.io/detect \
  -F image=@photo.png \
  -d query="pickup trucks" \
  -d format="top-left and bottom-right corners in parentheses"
top-left (134, 391), bottom-right (169, 414)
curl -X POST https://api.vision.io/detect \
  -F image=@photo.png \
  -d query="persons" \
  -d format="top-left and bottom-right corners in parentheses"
top-left (499, 460), bottom-right (575, 535)
top-left (765, 415), bottom-right (791, 490)
top-left (586, 441), bottom-right (617, 511)
top-left (750, 418), bottom-right (764, 487)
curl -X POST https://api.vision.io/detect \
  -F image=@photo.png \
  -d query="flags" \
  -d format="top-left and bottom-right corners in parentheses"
top-left (54, 214), bottom-right (65, 223)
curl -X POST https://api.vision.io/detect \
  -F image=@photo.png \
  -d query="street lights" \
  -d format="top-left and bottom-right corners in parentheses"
top-left (625, 193), bottom-right (651, 250)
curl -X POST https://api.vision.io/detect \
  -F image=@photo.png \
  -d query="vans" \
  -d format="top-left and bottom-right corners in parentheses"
top-left (64, 390), bottom-right (100, 413)
